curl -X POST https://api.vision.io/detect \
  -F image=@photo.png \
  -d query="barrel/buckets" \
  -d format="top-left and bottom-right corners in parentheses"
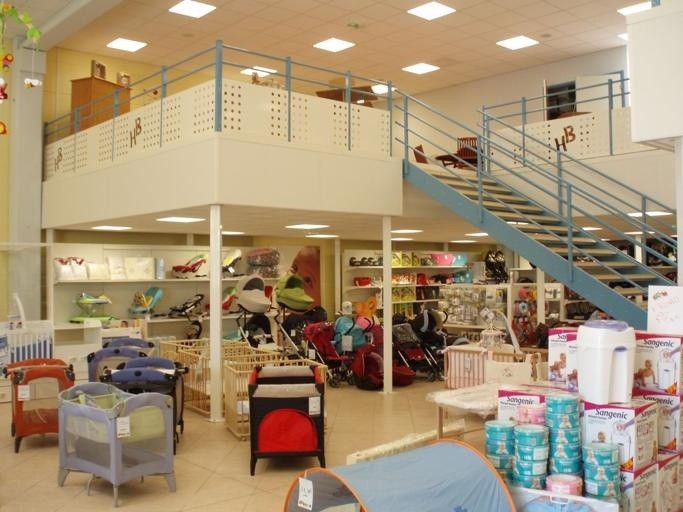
top-left (484, 392), bottom-right (621, 500)
top-left (576, 319), bottom-right (635, 404)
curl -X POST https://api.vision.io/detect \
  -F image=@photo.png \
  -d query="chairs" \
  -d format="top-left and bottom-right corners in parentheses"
top-left (414, 137), bottom-right (484, 169)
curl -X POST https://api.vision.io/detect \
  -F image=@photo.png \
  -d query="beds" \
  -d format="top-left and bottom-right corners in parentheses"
top-left (0, 322), bottom-right (327, 508)
top-left (444, 344), bottom-right (549, 389)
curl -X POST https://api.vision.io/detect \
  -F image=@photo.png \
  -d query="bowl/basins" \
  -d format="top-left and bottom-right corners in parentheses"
top-left (431, 253), bottom-right (468, 267)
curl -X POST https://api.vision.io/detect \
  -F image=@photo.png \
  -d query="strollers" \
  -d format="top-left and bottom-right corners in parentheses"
top-left (235, 310), bottom-right (469, 391)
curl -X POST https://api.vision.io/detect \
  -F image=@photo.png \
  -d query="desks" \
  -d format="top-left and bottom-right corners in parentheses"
top-left (433, 381), bottom-right (549, 440)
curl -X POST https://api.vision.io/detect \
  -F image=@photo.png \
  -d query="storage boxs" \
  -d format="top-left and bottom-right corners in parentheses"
top-left (498, 327), bottom-right (683, 512)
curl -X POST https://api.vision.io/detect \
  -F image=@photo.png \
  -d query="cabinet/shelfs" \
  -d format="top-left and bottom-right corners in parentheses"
top-left (70, 75), bottom-right (132, 132)
top-left (342, 249), bottom-right (565, 341)
top-left (46, 242), bottom-right (279, 380)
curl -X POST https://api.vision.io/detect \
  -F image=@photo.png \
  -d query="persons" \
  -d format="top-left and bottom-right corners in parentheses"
top-left (496, 352), bottom-right (678, 512)
top-left (284, 246), bottom-right (320, 314)
